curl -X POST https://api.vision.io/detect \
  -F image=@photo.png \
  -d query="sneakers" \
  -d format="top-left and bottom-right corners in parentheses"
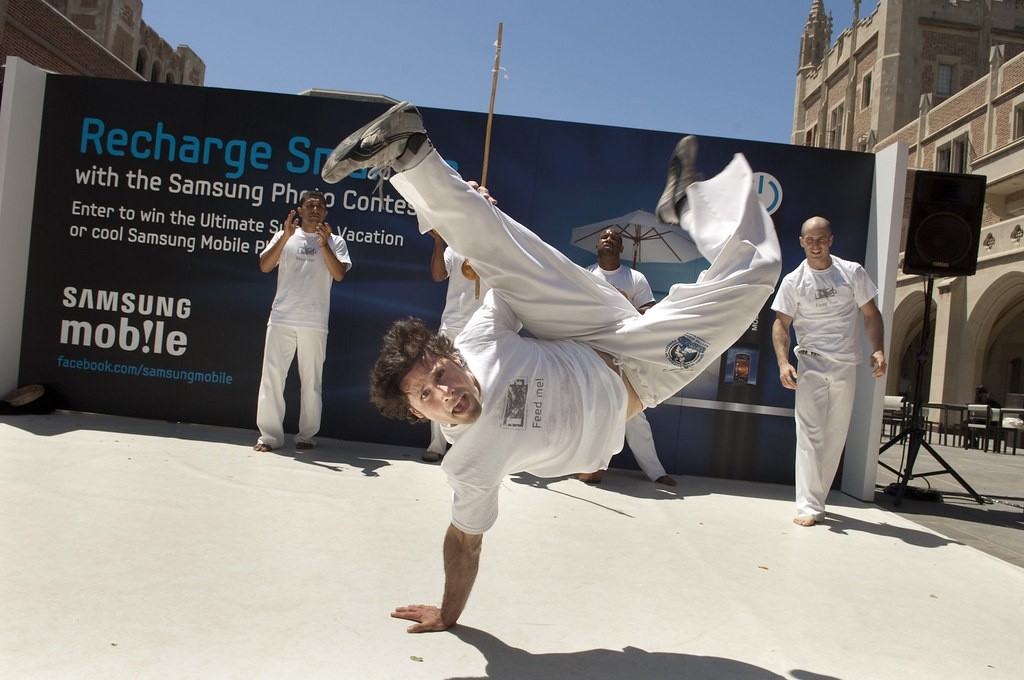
top-left (654, 134), bottom-right (700, 227)
top-left (321, 99), bottom-right (427, 192)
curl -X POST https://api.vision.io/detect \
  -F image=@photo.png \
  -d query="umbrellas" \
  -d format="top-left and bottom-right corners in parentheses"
top-left (570, 210), bottom-right (705, 270)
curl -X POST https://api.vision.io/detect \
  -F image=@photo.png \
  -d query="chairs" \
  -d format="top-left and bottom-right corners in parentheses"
top-left (881, 395), bottom-right (1024, 455)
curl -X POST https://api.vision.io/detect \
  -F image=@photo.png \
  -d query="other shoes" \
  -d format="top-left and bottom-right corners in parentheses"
top-left (422, 450), bottom-right (440, 462)
top-left (656, 475), bottom-right (677, 486)
top-left (578, 466), bottom-right (605, 483)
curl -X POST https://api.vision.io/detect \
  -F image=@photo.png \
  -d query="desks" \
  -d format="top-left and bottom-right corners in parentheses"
top-left (922, 403), bottom-right (967, 447)
top-left (972, 408), bottom-right (1019, 455)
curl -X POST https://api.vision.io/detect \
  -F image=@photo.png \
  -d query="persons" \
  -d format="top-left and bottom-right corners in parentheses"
top-left (770, 216), bottom-right (887, 526)
top-left (578, 227), bottom-right (678, 489)
top-left (320, 102), bottom-right (782, 632)
top-left (964, 385), bottom-right (1001, 449)
top-left (253, 190), bottom-right (352, 451)
top-left (423, 228), bottom-right (491, 461)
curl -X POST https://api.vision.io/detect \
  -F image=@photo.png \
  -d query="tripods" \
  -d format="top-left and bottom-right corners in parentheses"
top-left (877, 276), bottom-right (985, 507)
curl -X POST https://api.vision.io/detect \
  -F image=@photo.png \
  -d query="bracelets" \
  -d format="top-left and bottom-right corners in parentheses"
top-left (320, 244), bottom-right (326, 248)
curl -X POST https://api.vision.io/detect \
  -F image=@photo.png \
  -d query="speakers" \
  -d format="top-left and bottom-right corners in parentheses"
top-left (902, 169), bottom-right (987, 278)
